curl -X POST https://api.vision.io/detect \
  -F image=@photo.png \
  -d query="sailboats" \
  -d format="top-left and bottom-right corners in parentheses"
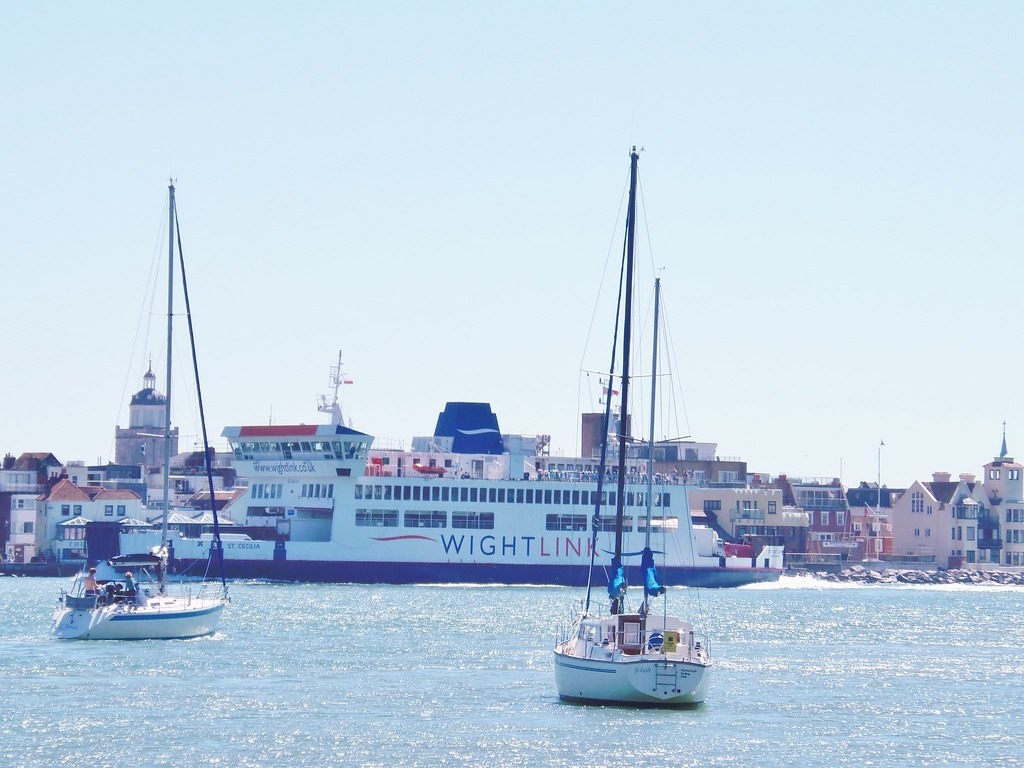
top-left (553, 145), bottom-right (714, 703)
top-left (50, 177), bottom-right (231, 640)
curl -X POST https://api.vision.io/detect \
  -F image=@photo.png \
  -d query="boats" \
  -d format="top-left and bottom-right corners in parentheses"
top-left (84, 424), bottom-right (785, 588)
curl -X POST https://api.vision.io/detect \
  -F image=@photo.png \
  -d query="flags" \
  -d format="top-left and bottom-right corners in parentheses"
top-left (603, 387), bottom-right (619, 396)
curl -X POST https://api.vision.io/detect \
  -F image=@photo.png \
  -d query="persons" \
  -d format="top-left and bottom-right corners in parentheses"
top-left (83, 568), bottom-right (102, 598)
top-left (100, 581), bottom-right (123, 595)
top-left (124, 571), bottom-right (138, 604)
